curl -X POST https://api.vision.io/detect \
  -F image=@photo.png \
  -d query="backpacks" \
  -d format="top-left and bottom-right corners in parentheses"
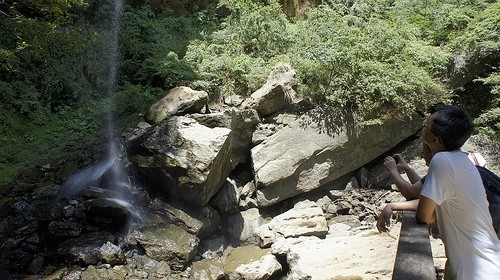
top-left (475, 164), bottom-right (500, 204)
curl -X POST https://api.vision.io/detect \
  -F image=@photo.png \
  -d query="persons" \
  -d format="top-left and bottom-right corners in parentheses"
top-left (382, 152), bottom-right (500, 230)
top-left (376, 104), bottom-right (500, 278)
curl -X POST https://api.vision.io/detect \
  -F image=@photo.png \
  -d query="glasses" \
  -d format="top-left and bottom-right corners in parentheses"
top-left (421, 118), bottom-right (442, 145)
